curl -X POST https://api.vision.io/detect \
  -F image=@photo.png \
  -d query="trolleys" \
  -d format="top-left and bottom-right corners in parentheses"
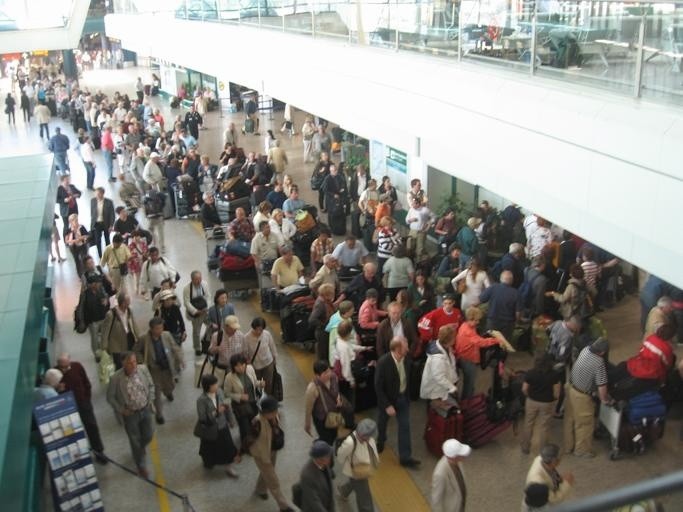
top-left (204, 224), bottom-right (234, 273)
top-left (593, 391), bottom-right (667, 460)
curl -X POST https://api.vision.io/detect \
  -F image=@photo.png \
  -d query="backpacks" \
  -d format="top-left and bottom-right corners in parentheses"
top-left (335, 432), bottom-right (357, 457)
top-left (568, 279), bottom-right (597, 320)
top-left (515, 267), bottom-right (541, 312)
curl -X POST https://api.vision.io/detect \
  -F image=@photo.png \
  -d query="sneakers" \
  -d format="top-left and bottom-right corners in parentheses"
top-left (240, 127), bottom-right (246, 135)
top-left (195, 350), bottom-right (201, 356)
top-left (253, 132), bottom-right (260, 135)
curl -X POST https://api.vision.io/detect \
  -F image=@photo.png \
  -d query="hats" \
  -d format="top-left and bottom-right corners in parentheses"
top-left (45, 368), bottom-right (63, 386)
top-left (588, 337), bottom-right (610, 354)
top-left (159, 289), bottom-right (177, 301)
top-left (224, 315), bottom-right (241, 331)
top-left (441, 438), bottom-right (472, 458)
top-left (260, 395), bottom-right (284, 414)
top-left (307, 440), bottom-right (335, 459)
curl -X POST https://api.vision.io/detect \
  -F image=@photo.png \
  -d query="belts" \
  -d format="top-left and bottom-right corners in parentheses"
top-left (569, 377), bottom-right (593, 397)
top-left (113, 266), bottom-right (120, 269)
top-left (216, 362), bottom-right (229, 370)
top-left (130, 401), bottom-right (149, 414)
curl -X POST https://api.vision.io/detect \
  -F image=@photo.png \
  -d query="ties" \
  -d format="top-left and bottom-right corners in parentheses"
top-left (366, 442), bottom-right (381, 471)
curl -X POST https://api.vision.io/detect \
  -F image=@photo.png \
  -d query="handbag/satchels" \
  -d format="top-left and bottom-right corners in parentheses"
top-left (309, 165), bottom-right (327, 189)
top-left (137, 229), bottom-right (153, 245)
top-left (187, 296), bottom-right (208, 319)
top-left (294, 204), bottom-right (318, 233)
top-left (427, 275), bottom-right (452, 294)
top-left (335, 359), bottom-right (342, 378)
top-left (72, 291), bottom-right (88, 334)
top-left (112, 248), bottom-right (128, 275)
top-left (127, 196), bottom-right (141, 208)
top-left (193, 361), bottom-right (226, 391)
top-left (175, 272), bottom-right (180, 284)
top-left (272, 363), bottom-right (283, 402)
top-left (271, 422), bottom-right (284, 451)
top-left (351, 462), bottom-right (374, 482)
top-left (285, 121), bottom-right (292, 130)
top-left (87, 230), bottom-right (100, 247)
top-left (97, 350), bottom-right (117, 385)
top-left (291, 482), bottom-right (302, 510)
top-left (324, 395), bottom-right (355, 429)
top-left (193, 397), bottom-right (219, 444)
top-left (200, 322), bottom-right (217, 357)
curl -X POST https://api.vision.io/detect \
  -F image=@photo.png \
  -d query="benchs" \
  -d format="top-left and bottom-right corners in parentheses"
top-left (501, 33), bottom-right (610, 71)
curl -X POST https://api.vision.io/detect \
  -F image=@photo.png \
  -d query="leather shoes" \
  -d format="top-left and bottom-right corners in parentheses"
top-left (139, 469), bottom-right (148, 478)
top-left (94, 452), bottom-right (108, 466)
top-left (255, 489), bottom-right (269, 501)
top-left (399, 458), bottom-right (421, 467)
top-left (155, 414), bottom-right (165, 424)
top-left (280, 505), bottom-right (296, 512)
top-left (226, 471), bottom-right (239, 480)
top-left (164, 392), bottom-right (174, 402)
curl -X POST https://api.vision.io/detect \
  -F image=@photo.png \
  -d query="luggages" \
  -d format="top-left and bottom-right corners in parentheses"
top-left (338, 349), bottom-right (379, 413)
top-left (217, 232), bottom-right (378, 347)
top-left (409, 359), bottom-right (426, 401)
top-left (510, 315), bottom-right (555, 357)
top-left (327, 196), bottom-right (347, 236)
top-left (416, 252), bottom-right (433, 277)
top-left (461, 394), bottom-right (512, 449)
top-left (244, 118), bottom-right (255, 134)
top-left (606, 359), bottom-right (668, 443)
top-left (170, 97), bottom-right (182, 109)
top-left (144, 85), bottom-right (150, 96)
top-left (422, 392), bottom-right (466, 459)
top-left (502, 382), bottom-right (526, 413)
top-left (144, 180), bottom-right (268, 220)
